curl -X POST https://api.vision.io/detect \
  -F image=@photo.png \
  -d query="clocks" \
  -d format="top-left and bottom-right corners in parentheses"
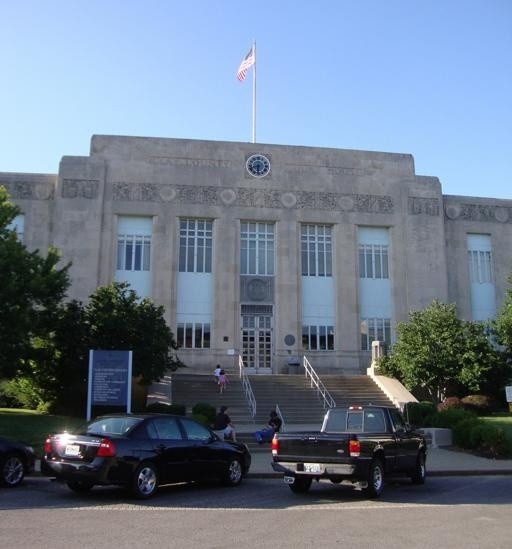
top-left (246, 154), bottom-right (271, 178)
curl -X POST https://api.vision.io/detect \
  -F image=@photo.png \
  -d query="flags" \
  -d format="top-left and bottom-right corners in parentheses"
top-left (237, 45), bottom-right (256, 81)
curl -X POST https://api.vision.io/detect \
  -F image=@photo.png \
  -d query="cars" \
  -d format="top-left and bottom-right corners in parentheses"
top-left (0, 438), bottom-right (35, 488)
top-left (41, 413), bottom-right (251, 500)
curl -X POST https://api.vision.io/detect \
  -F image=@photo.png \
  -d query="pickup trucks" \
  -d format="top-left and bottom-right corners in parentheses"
top-left (272, 405), bottom-right (428, 498)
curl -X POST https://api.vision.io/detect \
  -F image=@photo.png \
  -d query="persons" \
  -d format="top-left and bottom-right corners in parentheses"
top-left (213, 364), bottom-right (222, 384)
top-left (269, 412), bottom-right (281, 436)
top-left (218, 370), bottom-right (230, 393)
top-left (217, 406), bottom-right (237, 443)
